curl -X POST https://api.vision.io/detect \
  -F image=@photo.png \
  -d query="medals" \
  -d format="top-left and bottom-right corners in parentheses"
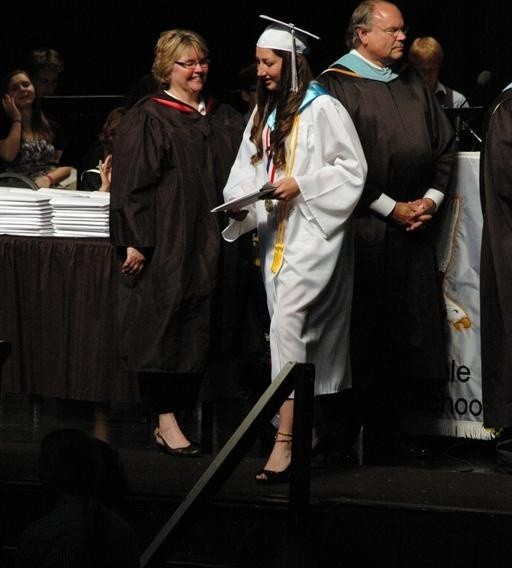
top-left (265, 199), bottom-right (274, 212)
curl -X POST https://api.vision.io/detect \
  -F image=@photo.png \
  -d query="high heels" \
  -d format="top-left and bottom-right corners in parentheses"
top-left (254, 457), bottom-right (292, 485)
top-left (153, 434), bottom-right (204, 457)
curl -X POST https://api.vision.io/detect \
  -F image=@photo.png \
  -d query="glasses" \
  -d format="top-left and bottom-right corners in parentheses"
top-left (374, 24), bottom-right (409, 35)
top-left (172, 56), bottom-right (212, 71)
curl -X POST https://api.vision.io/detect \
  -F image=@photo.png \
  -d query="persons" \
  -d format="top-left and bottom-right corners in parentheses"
top-left (409, 36), bottom-right (471, 108)
top-left (226, 58), bottom-right (259, 119)
top-left (474, 80), bottom-right (511, 455)
top-left (89, 29), bottom-right (247, 459)
top-left (221, 14), bottom-right (369, 484)
top-left (3, 43), bottom-right (129, 192)
top-left (312, 3), bottom-right (460, 471)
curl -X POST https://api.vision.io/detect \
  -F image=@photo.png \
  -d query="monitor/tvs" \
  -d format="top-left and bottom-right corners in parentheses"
top-left (440, 106), bottom-right (485, 150)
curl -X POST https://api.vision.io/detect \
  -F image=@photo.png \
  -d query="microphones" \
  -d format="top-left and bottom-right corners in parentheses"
top-left (460, 69), bottom-right (493, 107)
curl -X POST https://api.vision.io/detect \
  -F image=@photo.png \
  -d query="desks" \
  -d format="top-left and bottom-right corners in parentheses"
top-left (0, 236), bottom-right (127, 428)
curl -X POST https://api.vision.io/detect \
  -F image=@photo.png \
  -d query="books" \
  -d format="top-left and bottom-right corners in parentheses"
top-left (210, 182), bottom-right (279, 213)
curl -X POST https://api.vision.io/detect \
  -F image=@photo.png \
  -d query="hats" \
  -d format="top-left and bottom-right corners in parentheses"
top-left (256, 12), bottom-right (321, 93)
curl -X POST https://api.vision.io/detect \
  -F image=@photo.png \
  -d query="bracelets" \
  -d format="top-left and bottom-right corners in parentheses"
top-left (46, 175), bottom-right (52, 183)
top-left (13, 119), bottom-right (21, 123)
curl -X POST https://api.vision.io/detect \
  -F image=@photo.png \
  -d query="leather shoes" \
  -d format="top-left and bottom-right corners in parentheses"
top-left (401, 445), bottom-right (432, 457)
